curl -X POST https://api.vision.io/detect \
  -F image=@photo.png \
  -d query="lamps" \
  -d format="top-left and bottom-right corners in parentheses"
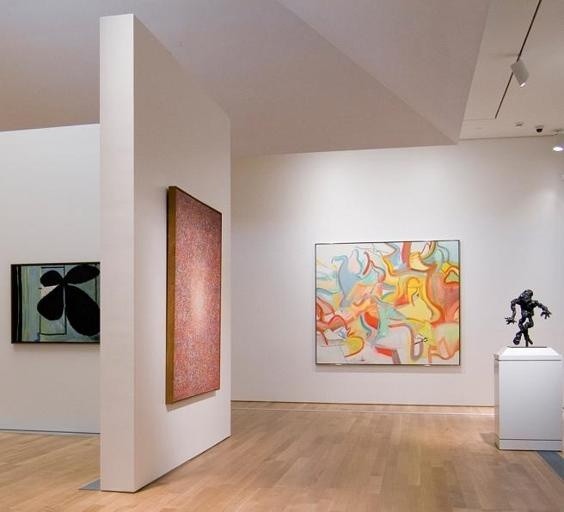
top-left (495, 0), bottom-right (542, 119)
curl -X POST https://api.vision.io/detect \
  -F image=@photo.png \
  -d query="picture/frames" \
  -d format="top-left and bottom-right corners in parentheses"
top-left (314, 242), bottom-right (461, 366)
top-left (10, 261), bottom-right (100, 345)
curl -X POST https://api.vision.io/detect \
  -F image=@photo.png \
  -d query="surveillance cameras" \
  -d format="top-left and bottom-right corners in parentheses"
top-left (535, 125), bottom-right (544, 133)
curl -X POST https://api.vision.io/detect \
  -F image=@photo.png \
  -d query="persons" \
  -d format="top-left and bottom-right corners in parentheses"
top-left (504, 289), bottom-right (554, 347)
